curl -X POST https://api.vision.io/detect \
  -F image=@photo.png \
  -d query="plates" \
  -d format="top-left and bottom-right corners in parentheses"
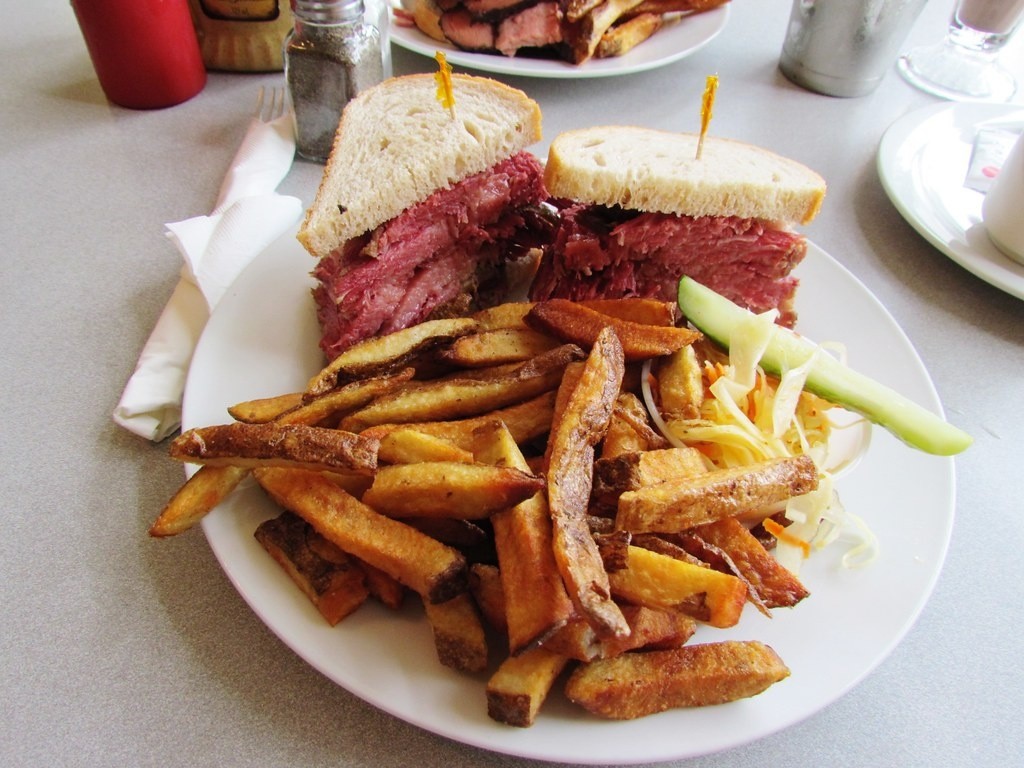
top-left (386, 0), bottom-right (732, 80)
top-left (875, 93), bottom-right (1024, 302)
top-left (178, 204), bottom-right (960, 765)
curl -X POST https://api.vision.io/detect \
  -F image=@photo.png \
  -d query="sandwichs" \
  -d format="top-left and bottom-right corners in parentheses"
top-left (295, 73), bottom-right (826, 362)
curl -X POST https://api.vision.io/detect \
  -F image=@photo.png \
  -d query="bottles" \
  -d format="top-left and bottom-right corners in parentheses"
top-left (281, 0), bottom-right (387, 165)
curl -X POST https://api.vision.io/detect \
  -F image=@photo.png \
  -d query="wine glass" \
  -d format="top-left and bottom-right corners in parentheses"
top-left (894, 1), bottom-right (1024, 105)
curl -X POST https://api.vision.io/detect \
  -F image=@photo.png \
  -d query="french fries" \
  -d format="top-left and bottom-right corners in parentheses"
top-left (402, 0), bottom-right (731, 64)
top-left (149, 298), bottom-right (819, 727)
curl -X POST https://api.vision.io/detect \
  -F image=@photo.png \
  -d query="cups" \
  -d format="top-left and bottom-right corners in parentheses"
top-left (777, 0), bottom-right (930, 98)
top-left (70, 0), bottom-right (209, 111)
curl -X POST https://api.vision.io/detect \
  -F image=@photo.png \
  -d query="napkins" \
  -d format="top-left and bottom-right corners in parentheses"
top-left (113, 108), bottom-right (301, 444)
